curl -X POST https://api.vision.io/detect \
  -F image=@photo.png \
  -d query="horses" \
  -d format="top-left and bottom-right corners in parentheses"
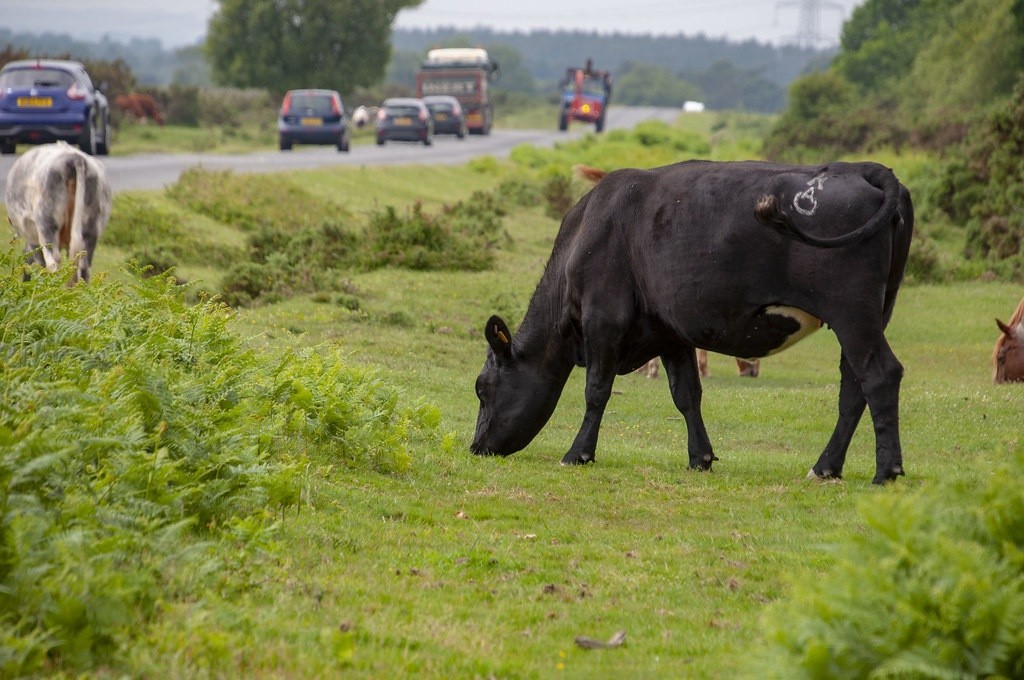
top-left (636, 347), bottom-right (760, 379)
top-left (990, 297), bottom-right (1024, 383)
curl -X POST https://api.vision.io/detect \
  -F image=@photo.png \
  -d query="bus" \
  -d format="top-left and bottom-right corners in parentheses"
top-left (413, 47), bottom-right (499, 136)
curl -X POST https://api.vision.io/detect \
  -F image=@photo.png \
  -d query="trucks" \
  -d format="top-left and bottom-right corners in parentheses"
top-left (561, 68), bottom-right (612, 133)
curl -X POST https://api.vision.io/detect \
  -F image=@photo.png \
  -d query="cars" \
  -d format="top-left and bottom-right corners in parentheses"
top-left (378, 97), bottom-right (434, 146)
top-left (425, 95), bottom-right (469, 139)
top-left (0, 59), bottom-right (113, 155)
top-left (277, 89), bottom-right (351, 152)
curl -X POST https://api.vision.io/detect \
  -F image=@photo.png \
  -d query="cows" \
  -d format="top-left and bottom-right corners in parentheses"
top-left (3, 140), bottom-right (113, 286)
top-left (470, 156), bottom-right (915, 484)
